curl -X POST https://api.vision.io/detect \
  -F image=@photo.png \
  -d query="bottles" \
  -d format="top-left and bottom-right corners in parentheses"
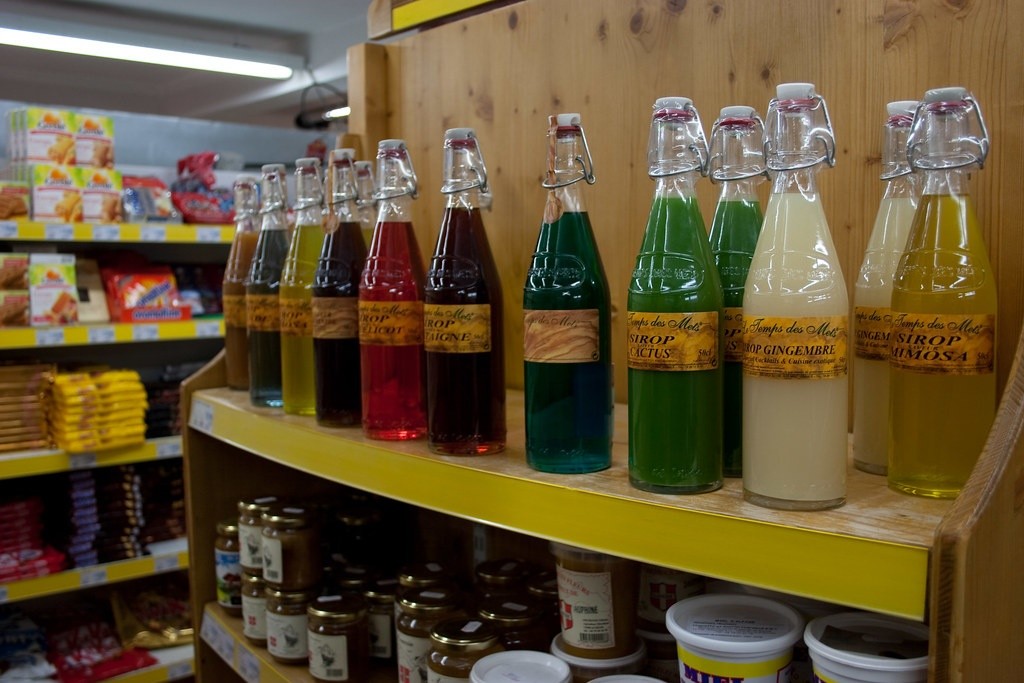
top-left (223, 149), bottom-right (376, 428)
top-left (214, 484), bottom-right (929, 683)
top-left (523, 111), bottom-right (613, 474)
top-left (709, 106), bottom-right (765, 478)
top-left (855, 100), bottom-right (922, 475)
top-left (741, 83), bottom-right (849, 512)
top-left (359, 139), bottom-right (429, 441)
top-left (626, 96), bottom-right (721, 496)
top-left (889, 85), bottom-right (995, 499)
top-left (422, 127), bottom-right (508, 457)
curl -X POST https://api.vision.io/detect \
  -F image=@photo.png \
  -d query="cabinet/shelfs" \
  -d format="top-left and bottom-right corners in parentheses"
top-left (179, 0), bottom-right (1024, 683)
top-left (0, 219), bottom-right (237, 683)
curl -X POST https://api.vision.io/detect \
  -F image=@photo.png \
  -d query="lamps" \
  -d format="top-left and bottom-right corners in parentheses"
top-left (0, 0), bottom-right (309, 84)
